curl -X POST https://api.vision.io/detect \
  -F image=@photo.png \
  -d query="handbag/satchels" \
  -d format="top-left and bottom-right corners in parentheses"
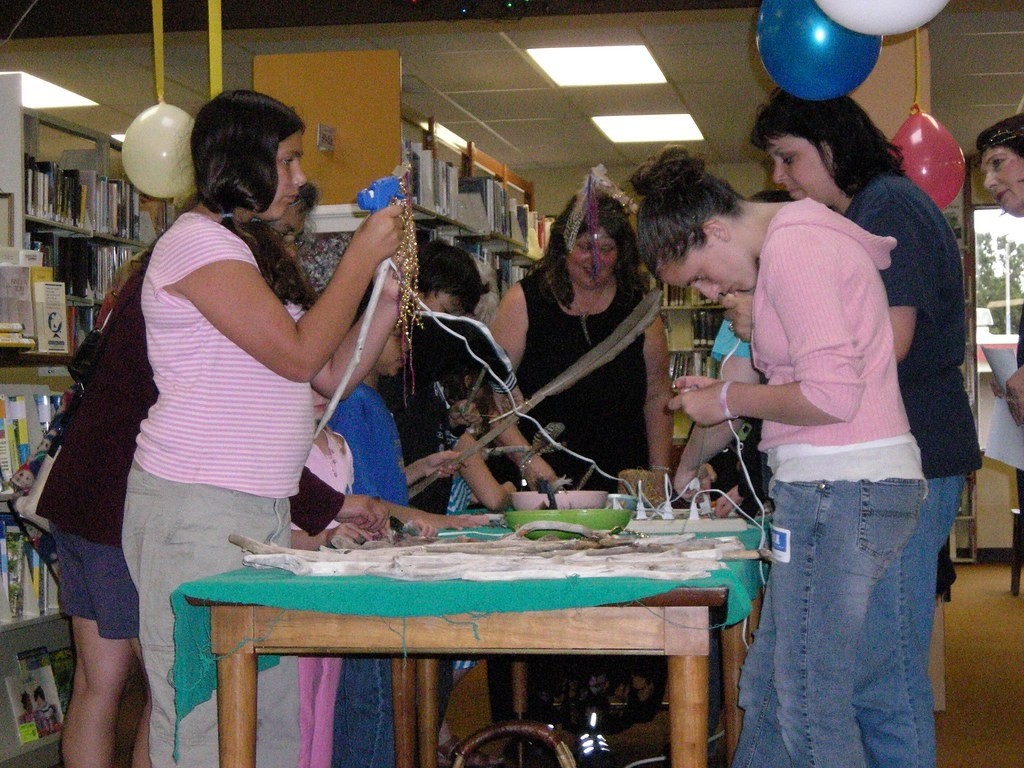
top-left (68, 328), bottom-right (104, 382)
top-left (23, 433), bottom-right (66, 534)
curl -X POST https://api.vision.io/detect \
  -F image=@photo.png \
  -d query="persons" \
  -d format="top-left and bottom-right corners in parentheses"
top-left (491, 193), bottom-right (673, 510)
top-left (120, 89), bottom-right (406, 768)
top-left (673, 190), bottom-right (794, 517)
top-left (290, 389), bottom-right (438, 768)
top-left (380, 241), bottom-right (556, 768)
top-left (328, 326), bottom-right (410, 768)
top-left (750, 91), bottom-right (983, 768)
top-left (34, 180), bottom-right (318, 768)
top-left (631, 144), bottom-right (930, 768)
top-left (976, 112), bottom-right (1024, 513)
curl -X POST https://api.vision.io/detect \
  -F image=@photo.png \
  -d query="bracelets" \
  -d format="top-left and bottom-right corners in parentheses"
top-left (720, 380), bottom-right (739, 418)
top-left (649, 465), bottom-right (671, 471)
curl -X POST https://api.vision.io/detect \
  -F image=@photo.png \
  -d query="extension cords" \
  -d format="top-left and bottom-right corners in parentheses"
top-left (622, 518), bottom-right (748, 533)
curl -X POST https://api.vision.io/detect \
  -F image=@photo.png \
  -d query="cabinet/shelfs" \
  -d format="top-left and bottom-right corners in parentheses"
top-left (631, 253), bottom-right (725, 467)
top-left (252, 48), bottom-right (555, 327)
top-left (0, 71), bottom-right (184, 768)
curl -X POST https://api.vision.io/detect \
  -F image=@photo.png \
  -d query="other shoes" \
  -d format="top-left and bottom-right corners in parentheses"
top-left (577, 734), bottom-right (615, 767)
top-left (508, 739), bottom-right (558, 768)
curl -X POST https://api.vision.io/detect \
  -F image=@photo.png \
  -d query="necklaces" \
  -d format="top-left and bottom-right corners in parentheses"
top-left (574, 288), bottom-right (604, 346)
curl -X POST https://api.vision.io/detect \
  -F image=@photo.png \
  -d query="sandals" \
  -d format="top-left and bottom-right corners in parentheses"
top-left (438, 742), bottom-right (505, 767)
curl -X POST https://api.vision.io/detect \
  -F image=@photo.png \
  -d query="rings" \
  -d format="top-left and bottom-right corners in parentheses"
top-left (1006, 397), bottom-right (1013, 402)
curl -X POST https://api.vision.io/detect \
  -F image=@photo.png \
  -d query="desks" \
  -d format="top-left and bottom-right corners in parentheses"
top-left (183, 509), bottom-right (772, 768)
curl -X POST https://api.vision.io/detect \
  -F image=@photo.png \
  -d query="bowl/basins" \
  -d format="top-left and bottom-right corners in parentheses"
top-left (511, 489), bottom-right (608, 510)
top-left (506, 510), bottom-right (631, 541)
top-left (603, 493), bottom-right (638, 510)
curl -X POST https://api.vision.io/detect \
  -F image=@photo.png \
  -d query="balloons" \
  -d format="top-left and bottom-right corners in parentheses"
top-left (122, 104), bottom-right (195, 198)
top-left (815, 0), bottom-right (950, 36)
top-left (756, 0), bottom-right (883, 101)
top-left (888, 111), bottom-right (966, 209)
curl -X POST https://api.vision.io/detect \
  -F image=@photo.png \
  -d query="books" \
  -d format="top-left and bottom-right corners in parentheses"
top-left (0, 149), bottom-right (181, 745)
top-left (406, 139), bottom-right (544, 297)
top-left (640, 271), bottom-right (727, 389)
